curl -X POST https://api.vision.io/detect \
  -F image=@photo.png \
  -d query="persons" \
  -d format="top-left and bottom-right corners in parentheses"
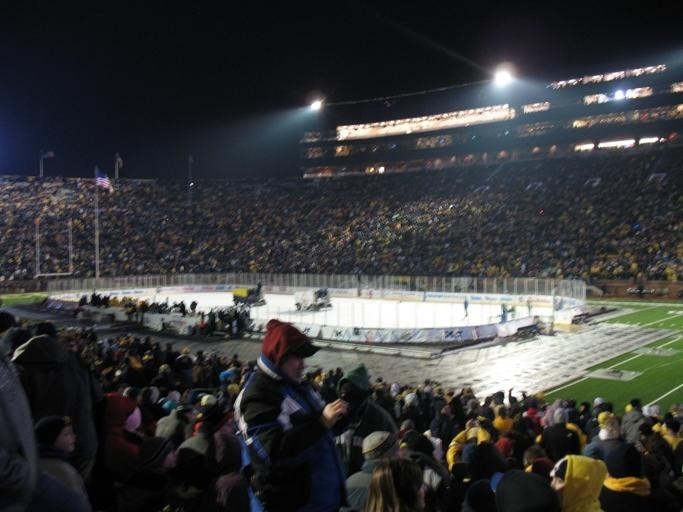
top-left (0, 142), bottom-right (683, 511)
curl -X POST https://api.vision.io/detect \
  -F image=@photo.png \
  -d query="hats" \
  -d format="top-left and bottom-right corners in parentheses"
top-left (360, 429), bottom-right (400, 461)
top-left (94, 333), bottom-right (171, 404)
top-left (1, 326), bottom-right (35, 357)
top-left (549, 458), bottom-right (568, 483)
top-left (597, 410), bottom-right (615, 428)
top-left (593, 397), bottom-right (604, 406)
top-left (32, 414), bottom-right (74, 447)
top-left (199, 394), bottom-right (220, 407)
top-left (218, 370), bottom-right (234, 382)
top-left (391, 379), bottom-right (455, 439)
top-left (293, 343), bottom-right (322, 359)
top-left (552, 408), bottom-right (569, 425)
top-left (182, 346), bottom-right (191, 356)
top-left (466, 398), bottom-right (480, 415)
